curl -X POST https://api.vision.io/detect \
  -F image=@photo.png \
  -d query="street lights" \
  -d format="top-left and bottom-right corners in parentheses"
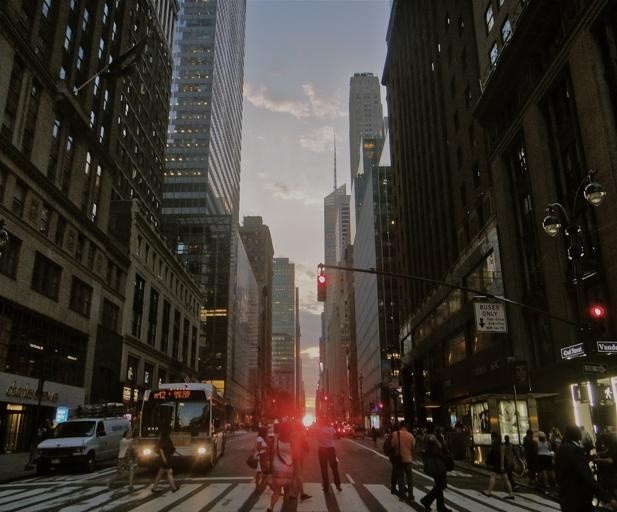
top-left (247, 455), bottom-right (258, 469)
top-left (485, 440), bottom-right (498, 466)
top-left (391, 454), bottom-right (402, 465)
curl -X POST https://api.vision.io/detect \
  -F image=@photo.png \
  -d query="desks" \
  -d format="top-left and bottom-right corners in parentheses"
top-left (536, 169), bottom-right (611, 441)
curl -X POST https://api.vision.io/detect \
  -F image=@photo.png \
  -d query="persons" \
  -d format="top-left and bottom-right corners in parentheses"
top-left (150, 423), bottom-right (180, 494)
top-left (23, 417), bottom-right (50, 472)
top-left (106, 430), bottom-right (140, 494)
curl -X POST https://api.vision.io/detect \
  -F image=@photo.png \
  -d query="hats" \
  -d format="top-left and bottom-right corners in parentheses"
top-left (335, 421), bottom-right (356, 436)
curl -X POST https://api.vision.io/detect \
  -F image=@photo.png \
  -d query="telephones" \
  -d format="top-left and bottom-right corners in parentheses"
top-left (581, 362), bottom-right (608, 374)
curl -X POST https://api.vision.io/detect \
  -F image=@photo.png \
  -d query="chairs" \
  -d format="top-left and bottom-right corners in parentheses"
top-left (421, 497), bottom-right (432, 512)
top-left (438, 507), bottom-right (452, 512)
top-left (301, 494), bottom-right (312, 499)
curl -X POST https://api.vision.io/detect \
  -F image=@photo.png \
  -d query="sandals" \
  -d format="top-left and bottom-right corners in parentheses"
top-left (134, 383), bottom-right (230, 473)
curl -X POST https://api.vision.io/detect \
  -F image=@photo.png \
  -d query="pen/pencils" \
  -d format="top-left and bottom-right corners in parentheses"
top-left (35, 417), bottom-right (133, 479)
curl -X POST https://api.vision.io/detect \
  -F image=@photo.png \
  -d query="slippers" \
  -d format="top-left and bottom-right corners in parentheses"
top-left (383, 435), bottom-right (395, 461)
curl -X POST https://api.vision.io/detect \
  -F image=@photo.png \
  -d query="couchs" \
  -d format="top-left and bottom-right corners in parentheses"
top-left (590, 303), bottom-right (605, 323)
top-left (315, 273), bottom-right (329, 302)
